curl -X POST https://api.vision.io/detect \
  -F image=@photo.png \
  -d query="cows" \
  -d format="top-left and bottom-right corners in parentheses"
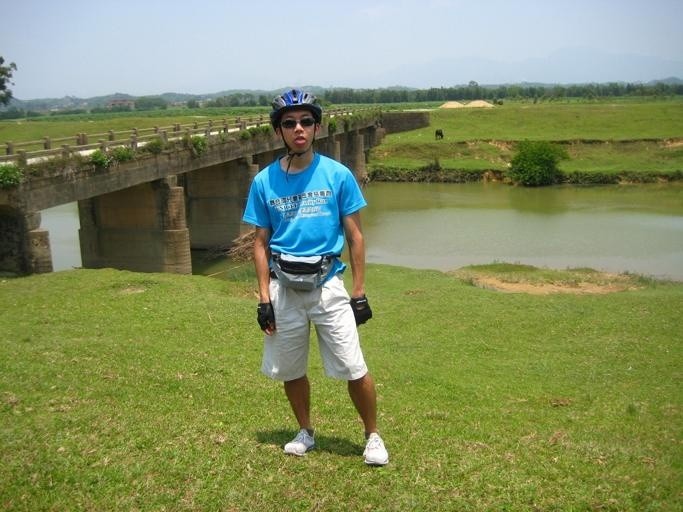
top-left (435, 129), bottom-right (444, 140)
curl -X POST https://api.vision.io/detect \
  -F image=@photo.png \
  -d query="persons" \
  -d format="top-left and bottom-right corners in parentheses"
top-left (243, 88), bottom-right (388, 466)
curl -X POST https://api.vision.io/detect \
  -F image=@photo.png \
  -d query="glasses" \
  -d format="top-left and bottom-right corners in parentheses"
top-left (279, 117), bottom-right (315, 129)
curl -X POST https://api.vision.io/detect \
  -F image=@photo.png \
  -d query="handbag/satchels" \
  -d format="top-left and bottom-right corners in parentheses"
top-left (271, 249), bottom-right (334, 291)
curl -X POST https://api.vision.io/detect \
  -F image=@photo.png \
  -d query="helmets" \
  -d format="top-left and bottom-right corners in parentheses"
top-left (270, 88), bottom-right (322, 131)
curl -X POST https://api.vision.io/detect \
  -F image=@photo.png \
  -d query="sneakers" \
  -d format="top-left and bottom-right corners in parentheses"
top-left (363, 432), bottom-right (389, 465)
top-left (283, 428), bottom-right (315, 456)
